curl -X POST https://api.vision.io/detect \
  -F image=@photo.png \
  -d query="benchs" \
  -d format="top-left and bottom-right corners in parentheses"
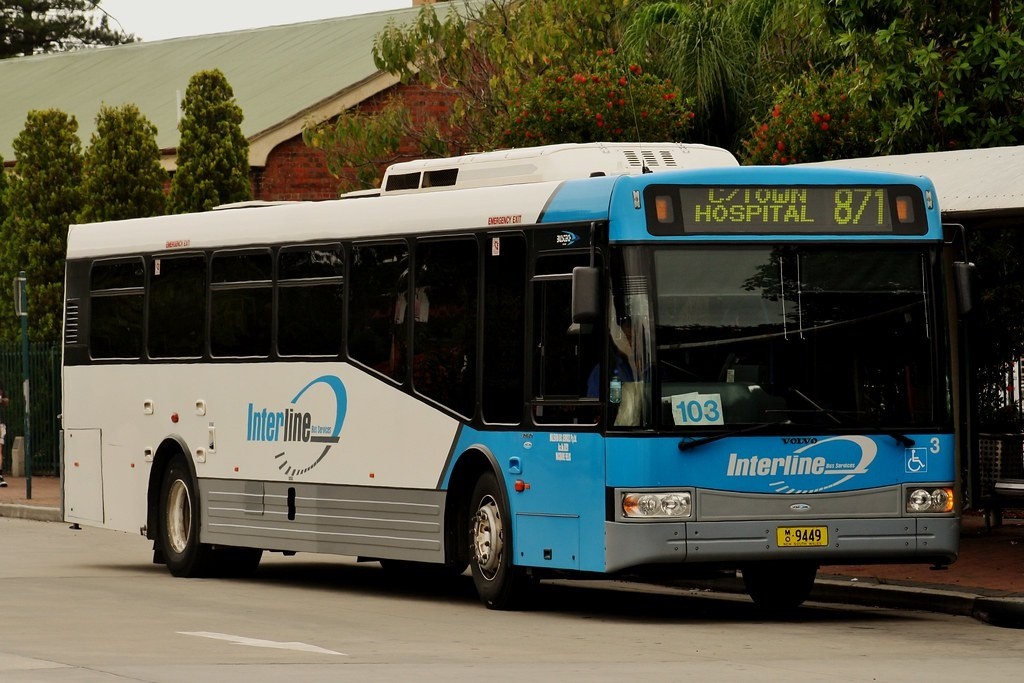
top-left (977, 433), bottom-right (1024, 532)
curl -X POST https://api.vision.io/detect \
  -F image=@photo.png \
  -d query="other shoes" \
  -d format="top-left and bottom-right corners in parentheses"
top-left (0, 475), bottom-right (8, 487)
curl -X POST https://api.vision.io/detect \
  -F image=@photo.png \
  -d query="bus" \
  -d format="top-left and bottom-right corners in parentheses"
top-left (59, 141), bottom-right (965, 610)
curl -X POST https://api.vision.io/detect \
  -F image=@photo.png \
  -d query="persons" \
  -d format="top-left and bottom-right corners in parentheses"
top-left (387, 253), bottom-right (434, 384)
top-left (0, 389), bottom-right (8, 487)
